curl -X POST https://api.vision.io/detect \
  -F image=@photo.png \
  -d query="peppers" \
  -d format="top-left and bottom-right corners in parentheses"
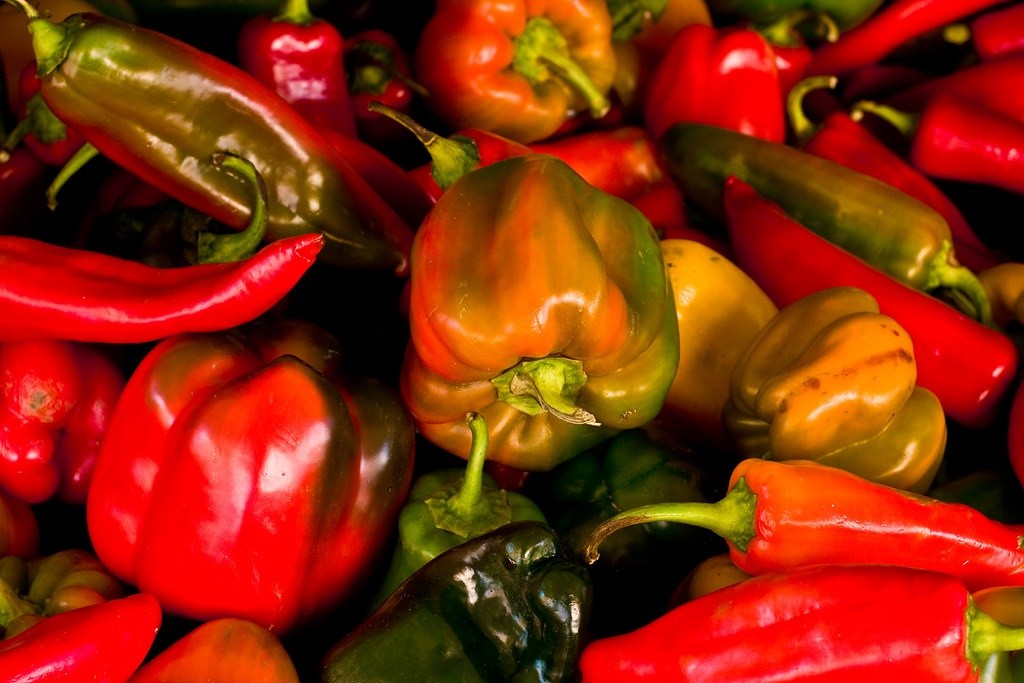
top-left (0, 0), bottom-right (1024, 683)
